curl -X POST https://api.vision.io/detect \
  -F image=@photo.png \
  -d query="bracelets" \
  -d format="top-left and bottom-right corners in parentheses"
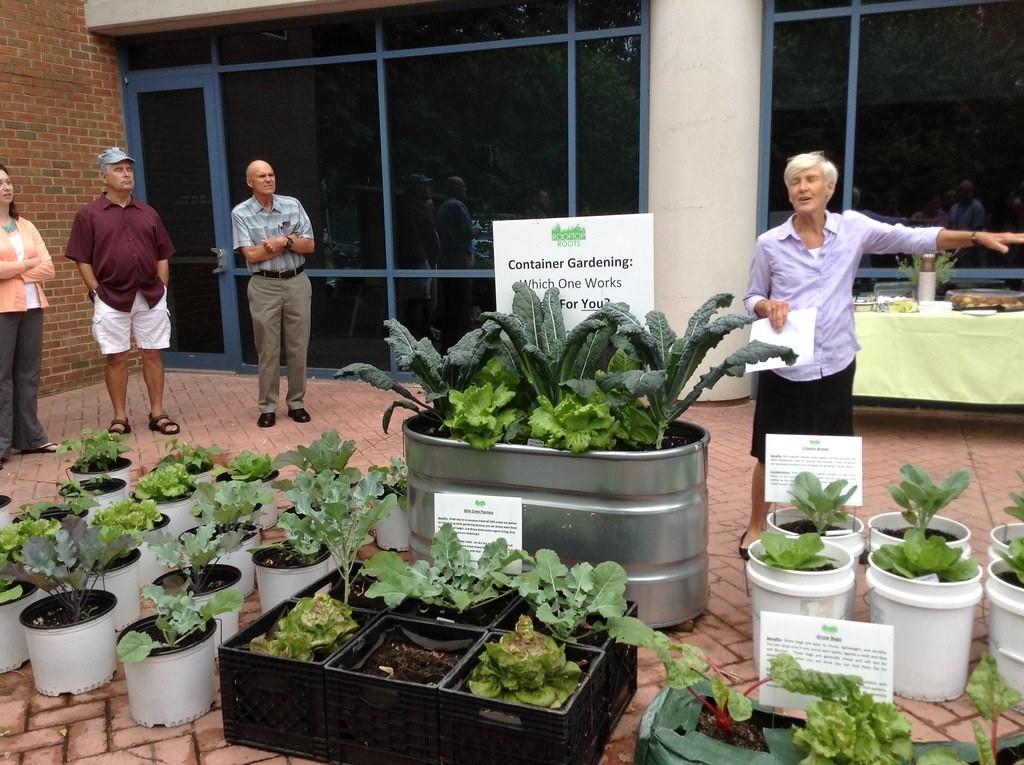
top-left (972, 230), bottom-right (981, 246)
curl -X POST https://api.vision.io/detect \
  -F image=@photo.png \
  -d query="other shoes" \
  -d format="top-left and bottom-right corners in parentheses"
top-left (288, 408), bottom-right (311, 422)
top-left (258, 412), bottom-right (276, 427)
top-left (738, 529), bottom-right (751, 560)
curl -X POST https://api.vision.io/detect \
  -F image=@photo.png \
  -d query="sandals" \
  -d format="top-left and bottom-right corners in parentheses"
top-left (108, 415), bottom-right (131, 435)
top-left (149, 411), bottom-right (180, 434)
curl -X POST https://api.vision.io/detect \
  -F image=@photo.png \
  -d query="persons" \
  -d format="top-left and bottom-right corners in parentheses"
top-left (854, 181), bottom-right (1024, 295)
top-left (0, 163), bottom-right (62, 471)
top-left (396, 173), bottom-right (549, 358)
top-left (231, 160), bottom-right (315, 427)
top-left (65, 150), bottom-right (180, 435)
top-left (738, 152), bottom-right (1024, 563)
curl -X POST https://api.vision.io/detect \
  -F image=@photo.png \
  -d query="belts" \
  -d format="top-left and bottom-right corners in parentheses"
top-left (253, 264), bottom-right (304, 279)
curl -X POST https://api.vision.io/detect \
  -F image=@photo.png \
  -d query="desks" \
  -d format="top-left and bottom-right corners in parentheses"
top-left (850, 296), bottom-right (1024, 414)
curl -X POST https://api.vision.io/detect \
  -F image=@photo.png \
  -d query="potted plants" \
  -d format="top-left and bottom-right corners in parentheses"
top-left (0, 283), bottom-right (1024, 765)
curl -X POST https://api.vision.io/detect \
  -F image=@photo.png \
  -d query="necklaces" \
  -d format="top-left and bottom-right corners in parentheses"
top-left (1, 217), bottom-right (16, 233)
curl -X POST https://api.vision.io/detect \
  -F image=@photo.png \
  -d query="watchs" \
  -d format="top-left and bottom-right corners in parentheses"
top-left (90, 289), bottom-right (98, 298)
top-left (285, 236), bottom-right (293, 249)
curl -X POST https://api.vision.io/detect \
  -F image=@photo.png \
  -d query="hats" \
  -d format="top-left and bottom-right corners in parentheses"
top-left (98, 146), bottom-right (136, 169)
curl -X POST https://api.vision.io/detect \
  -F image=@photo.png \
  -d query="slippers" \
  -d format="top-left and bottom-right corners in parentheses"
top-left (21, 442), bottom-right (59, 452)
